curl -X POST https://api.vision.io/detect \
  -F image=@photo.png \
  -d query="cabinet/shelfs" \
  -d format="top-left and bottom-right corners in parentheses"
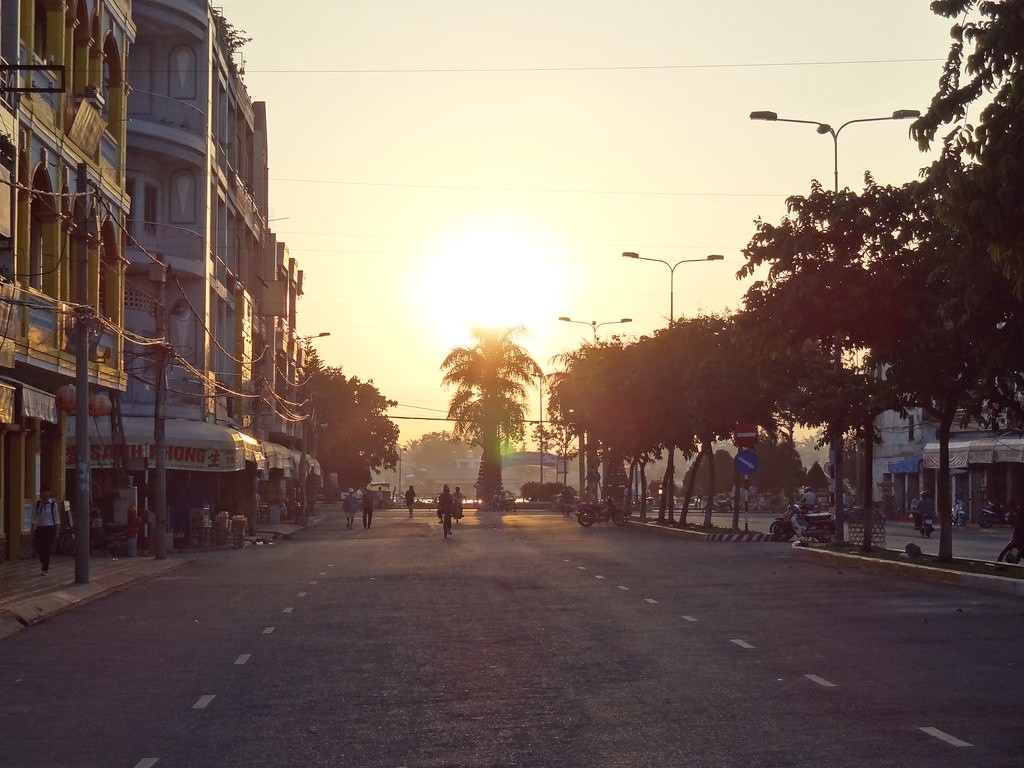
top-left (189, 506), bottom-right (211, 546)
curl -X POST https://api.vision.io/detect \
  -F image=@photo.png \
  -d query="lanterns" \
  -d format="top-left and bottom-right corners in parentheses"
top-left (89, 394), bottom-right (112, 416)
top-left (55, 386), bottom-right (76, 412)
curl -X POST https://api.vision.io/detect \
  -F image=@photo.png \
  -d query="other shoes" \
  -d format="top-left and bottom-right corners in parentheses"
top-left (350, 526), bottom-right (353, 529)
top-left (438, 521), bottom-right (443, 523)
top-left (346, 524), bottom-right (349, 529)
top-left (448, 532), bottom-right (452, 535)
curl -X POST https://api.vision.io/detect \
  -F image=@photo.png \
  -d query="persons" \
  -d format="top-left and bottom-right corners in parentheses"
top-left (561, 485), bottom-right (572, 497)
top-left (405, 486), bottom-right (416, 518)
top-left (437, 487), bottom-right (453, 523)
top-left (30, 484), bottom-right (60, 576)
top-left (802, 486), bottom-right (817, 510)
top-left (913, 491), bottom-right (931, 530)
top-left (363, 490), bottom-right (374, 529)
top-left (343, 488), bottom-right (358, 529)
top-left (452, 487), bottom-right (465, 518)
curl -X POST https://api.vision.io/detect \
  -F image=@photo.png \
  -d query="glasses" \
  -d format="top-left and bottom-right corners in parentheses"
top-left (443, 489), bottom-right (449, 492)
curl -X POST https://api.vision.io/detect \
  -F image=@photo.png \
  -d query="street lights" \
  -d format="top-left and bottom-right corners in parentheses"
top-left (748, 110), bottom-right (927, 546)
top-left (621, 250), bottom-right (732, 519)
top-left (532, 374), bottom-right (545, 484)
top-left (558, 316), bottom-right (631, 344)
top-left (399, 448), bottom-right (407, 496)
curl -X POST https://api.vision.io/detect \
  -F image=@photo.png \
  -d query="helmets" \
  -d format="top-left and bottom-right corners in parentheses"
top-left (921, 491), bottom-right (929, 499)
top-left (805, 486), bottom-right (812, 491)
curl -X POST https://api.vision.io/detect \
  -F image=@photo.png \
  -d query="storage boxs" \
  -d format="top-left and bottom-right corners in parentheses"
top-left (212, 517), bottom-right (247, 549)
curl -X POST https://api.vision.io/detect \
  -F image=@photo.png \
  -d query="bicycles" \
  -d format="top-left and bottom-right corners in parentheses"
top-left (440, 510), bottom-right (451, 538)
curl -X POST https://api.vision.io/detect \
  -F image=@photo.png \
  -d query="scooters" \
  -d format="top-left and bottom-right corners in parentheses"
top-left (59, 506), bottom-right (129, 559)
top-left (978, 499), bottom-right (1018, 528)
top-left (995, 515), bottom-right (1024, 564)
top-left (453, 497), bottom-right (466, 524)
top-left (768, 499), bottom-right (836, 544)
top-left (952, 494), bottom-right (975, 527)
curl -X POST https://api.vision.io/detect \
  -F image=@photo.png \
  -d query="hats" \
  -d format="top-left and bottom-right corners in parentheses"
top-left (348, 487), bottom-right (354, 493)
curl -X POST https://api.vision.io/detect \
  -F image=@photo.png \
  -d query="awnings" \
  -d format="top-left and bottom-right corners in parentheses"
top-left (923, 439), bottom-right (1024, 468)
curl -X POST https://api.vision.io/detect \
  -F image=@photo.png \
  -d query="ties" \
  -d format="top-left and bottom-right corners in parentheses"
top-left (39, 502), bottom-right (50, 514)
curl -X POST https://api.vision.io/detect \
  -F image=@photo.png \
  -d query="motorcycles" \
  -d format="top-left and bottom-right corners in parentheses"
top-left (913, 508), bottom-right (936, 539)
top-left (576, 496), bottom-right (628, 528)
